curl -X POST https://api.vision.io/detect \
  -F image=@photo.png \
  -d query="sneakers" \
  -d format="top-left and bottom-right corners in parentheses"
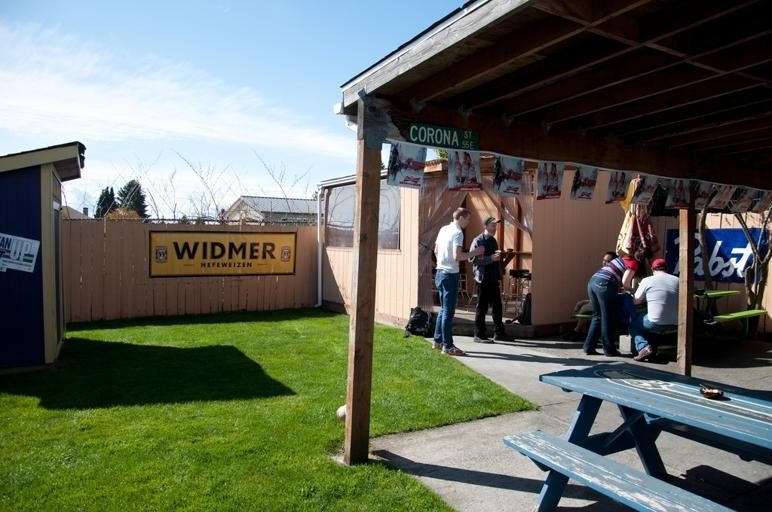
top-left (432, 341), bottom-right (443, 349)
top-left (443, 345), bottom-right (465, 355)
top-left (474, 336), bottom-right (493, 343)
top-left (493, 332), bottom-right (514, 341)
top-left (556, 329), bottom-right (577, 342)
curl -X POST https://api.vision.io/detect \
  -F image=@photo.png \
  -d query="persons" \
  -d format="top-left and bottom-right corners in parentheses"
top-left (388, 143), bottom-right (476, 184)
top-left (633, 177), bottom-right (771, 213)
top-left (493, 157), bottom-right (559, 193)
top-left (432, 206), bottom-right (486, 357)
top-left (627, 256), bottom-right (681, 362)
top-left (469, 215), bottom-right (517, 344)
top-left (572, 168), bottom-right (626, 200)
top-left (602, 250), bottom-right (639, 335)
top-left (582, 247), bottom-right (653, 359)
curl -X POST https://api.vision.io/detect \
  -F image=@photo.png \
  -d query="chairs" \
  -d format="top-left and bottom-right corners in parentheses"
top-left (429, 264), bottom-right (532, 318)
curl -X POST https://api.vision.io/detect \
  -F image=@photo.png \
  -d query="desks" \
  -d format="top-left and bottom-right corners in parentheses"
top-left (535, 363), bottom-right (772, 512)
top-left (694, 287), bottom-right (740, 316)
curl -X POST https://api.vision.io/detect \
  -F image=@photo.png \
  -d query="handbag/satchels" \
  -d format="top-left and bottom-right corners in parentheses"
top-left (403, 307), bottom-right (438, 338)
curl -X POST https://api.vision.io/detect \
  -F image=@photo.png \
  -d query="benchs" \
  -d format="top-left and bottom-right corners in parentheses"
top-left (571, 307), bottom-right (647, 321)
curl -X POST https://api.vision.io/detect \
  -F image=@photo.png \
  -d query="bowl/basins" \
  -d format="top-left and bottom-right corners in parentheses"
top-left (700, 387), bottom-right (724, 400)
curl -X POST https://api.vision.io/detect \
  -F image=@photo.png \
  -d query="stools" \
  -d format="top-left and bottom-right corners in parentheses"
top-left (702, 308), bottom-right (769, 328)
top-left (500, 427), bottom-right (733, 510)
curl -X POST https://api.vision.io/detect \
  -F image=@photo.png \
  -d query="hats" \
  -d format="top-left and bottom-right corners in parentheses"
top-left (652, 259), bottom-right (667, 270)
top-left (483, 215), bottom-right (502, 225)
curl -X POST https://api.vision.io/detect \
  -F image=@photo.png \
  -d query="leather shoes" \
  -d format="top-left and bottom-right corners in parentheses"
top-left (634, 346), bottom-right (654, 361)
